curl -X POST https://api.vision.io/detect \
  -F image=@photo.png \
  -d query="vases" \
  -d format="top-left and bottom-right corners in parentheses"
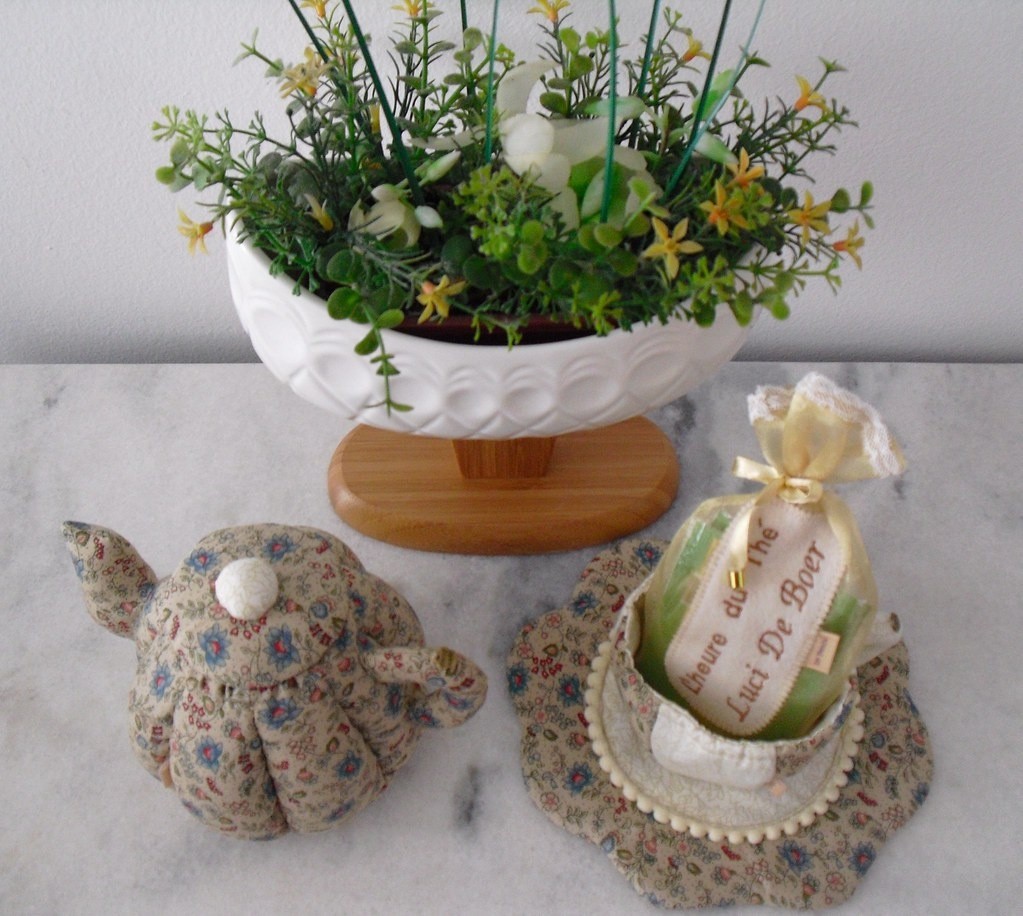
top-left (225, 190), bottom-right (766, 557)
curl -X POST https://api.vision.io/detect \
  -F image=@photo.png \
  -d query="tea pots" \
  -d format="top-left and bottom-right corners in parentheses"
top-left (62, 519), bottom-right (486, 842)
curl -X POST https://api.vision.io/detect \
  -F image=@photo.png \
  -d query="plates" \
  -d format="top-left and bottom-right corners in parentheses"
top-left (502, 538), bottom-right (933, 911)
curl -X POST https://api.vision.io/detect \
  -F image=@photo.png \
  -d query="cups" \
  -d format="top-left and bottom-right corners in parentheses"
top-left (614, 570), bottom-right (855, 781)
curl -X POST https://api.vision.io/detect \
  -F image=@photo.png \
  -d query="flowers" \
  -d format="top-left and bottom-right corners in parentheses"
top-left (152, 0), bottom-right (875, 419)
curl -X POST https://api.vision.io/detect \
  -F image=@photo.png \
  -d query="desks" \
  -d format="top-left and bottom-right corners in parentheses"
top-left (0, 362), bottom-right (1023, 916)
